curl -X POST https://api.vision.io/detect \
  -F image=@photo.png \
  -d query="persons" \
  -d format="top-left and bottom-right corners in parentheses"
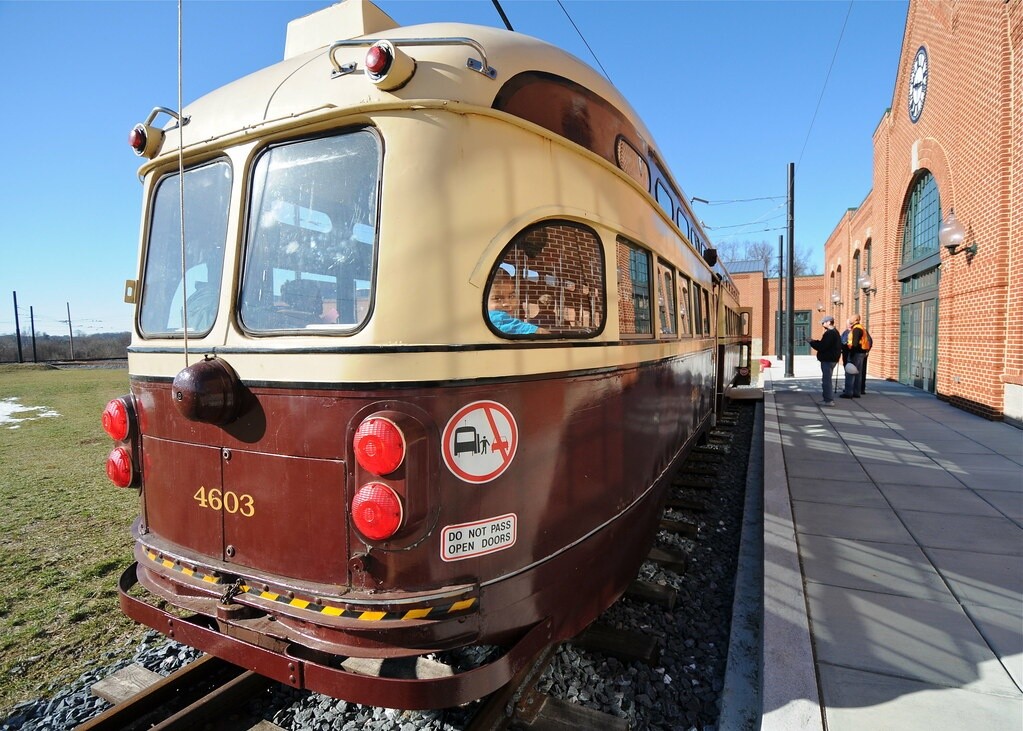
top-left (487, 267), bottom-right (552, 334)
top-left (181, 241), bottom-right (323, 331)
top-left (839, 314), bottom-right (873, 398)
top-left (806, 316), bottom-right (842, 406)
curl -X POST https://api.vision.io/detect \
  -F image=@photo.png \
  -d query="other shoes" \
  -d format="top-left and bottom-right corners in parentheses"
top-left (861, 388), bottom-right (866, 395)
top-left (839, 393), bottom-right (861, 399)
top-left (816, 399), bottom-right (835, 407)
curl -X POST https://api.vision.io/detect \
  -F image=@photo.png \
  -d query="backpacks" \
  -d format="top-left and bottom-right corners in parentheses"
top-left (181, 280), bottom-right (220, 332)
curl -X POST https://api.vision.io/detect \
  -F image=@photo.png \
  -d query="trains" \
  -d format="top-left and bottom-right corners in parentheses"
top-left (102, 0), bottom-right (754, 709)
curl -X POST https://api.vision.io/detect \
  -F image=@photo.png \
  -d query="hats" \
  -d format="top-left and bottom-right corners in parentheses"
top-left (818, 316), bottom-right (834, 323)
top-left (844, 363), bottom-right (859, 375)
